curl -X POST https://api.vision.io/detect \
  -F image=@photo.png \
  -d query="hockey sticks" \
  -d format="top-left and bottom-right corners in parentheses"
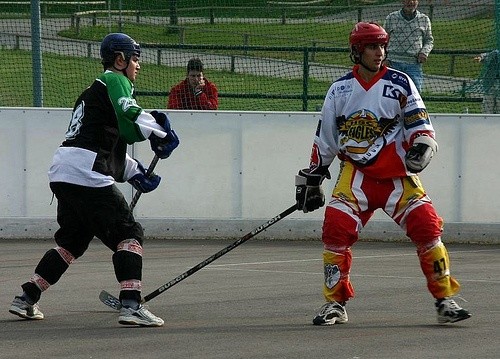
top-left (127, 154), bottom-right (161, 210)
top-left (98, 202), bottom-right (298, 312)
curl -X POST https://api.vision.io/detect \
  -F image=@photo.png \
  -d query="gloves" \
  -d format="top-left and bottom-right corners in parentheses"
top-left (294, 168), bottom-right (332, 213)
top-left (147, 109), bottom-right (180, 159)
top-left (403, 134), bottom-right (438, 173)
top-left (127, 158), bottom-right (162, 194)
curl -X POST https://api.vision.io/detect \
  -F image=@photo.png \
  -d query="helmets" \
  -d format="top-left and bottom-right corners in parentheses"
top-left (100, 33), bottom-right (141, 58)
top-left (349, 21), bottom-right (389, 44)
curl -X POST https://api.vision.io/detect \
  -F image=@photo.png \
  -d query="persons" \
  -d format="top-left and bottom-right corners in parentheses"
top-left (9, 33), bottom-right (179, 327)
top-left (474, 49), bottom-right (500, 114)
top-left (295, 23), bottom-right (471, 325)
top-left (381, 0), bottom-right (434, 96)
top-left (168, 58), bottom-right (218, 110)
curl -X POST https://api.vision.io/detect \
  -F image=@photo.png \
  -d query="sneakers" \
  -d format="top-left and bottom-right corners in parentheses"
top-left (435, 296), bottom-right (472, 324)
top-left (312, 300), bottom-right (348, 326)
top-left (118, 304), bottom-right (165, 328)
top-left (8, 296), bottom-right (44, 320)
